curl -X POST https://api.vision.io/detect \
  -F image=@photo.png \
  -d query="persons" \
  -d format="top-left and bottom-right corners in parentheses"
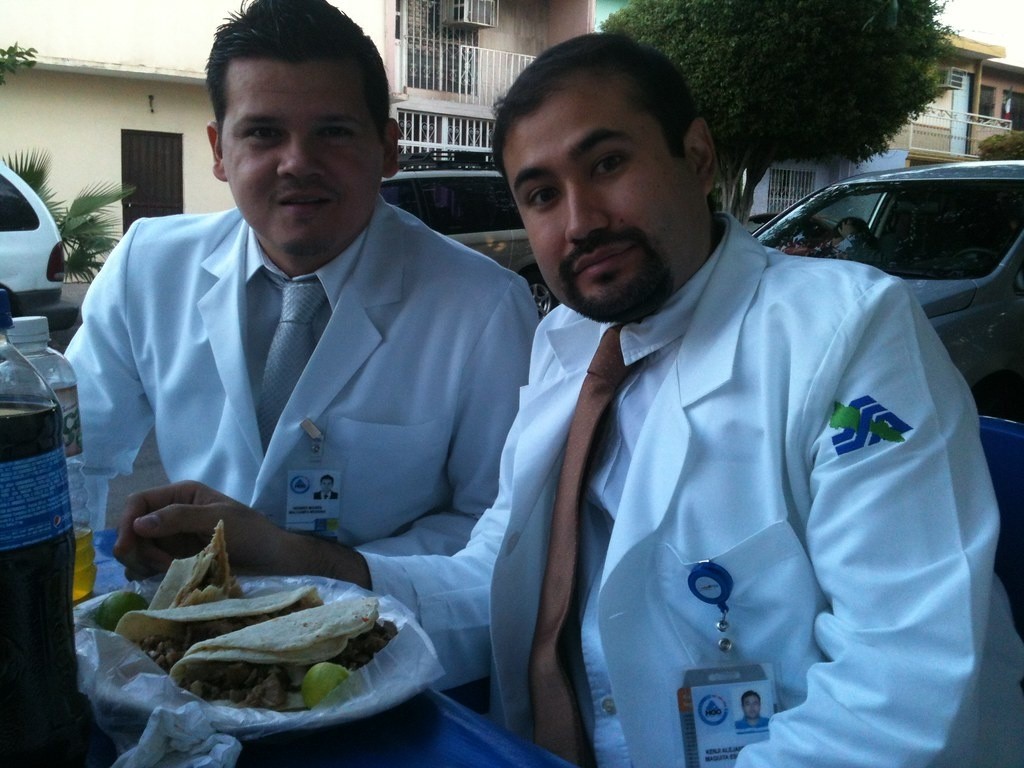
top-left (734, 690), bottom-right (769, 734)
top-left (313, 474), bottom-right (337, 500)
top-left (63, 0), bottom-right (538, 610)
top-left (834, 218), bottom-right (857, 251)
top-left (114, 33), bottom-right (1024, 768)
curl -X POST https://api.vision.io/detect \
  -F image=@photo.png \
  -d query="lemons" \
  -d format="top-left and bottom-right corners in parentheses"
top-left (300, 662), bottom-right (351, 709)
top-left (96, 590), bottom-right (148, 632)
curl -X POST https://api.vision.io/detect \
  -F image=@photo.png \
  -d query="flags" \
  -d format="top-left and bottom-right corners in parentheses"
top-left (1004, 87), bottom-right (1012, 119)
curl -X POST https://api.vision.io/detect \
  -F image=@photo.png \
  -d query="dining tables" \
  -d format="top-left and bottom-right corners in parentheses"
top-left (77, 528), bottom-right (582, 768)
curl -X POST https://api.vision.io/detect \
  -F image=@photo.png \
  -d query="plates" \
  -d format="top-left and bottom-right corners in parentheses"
top-left (71, 578), bottom-right (444, 730)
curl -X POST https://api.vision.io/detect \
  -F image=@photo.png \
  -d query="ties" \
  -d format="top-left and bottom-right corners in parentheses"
top-left (259, 269), bottom-right (327, 452)
top-left (531, 325), bottom-right (644, 768)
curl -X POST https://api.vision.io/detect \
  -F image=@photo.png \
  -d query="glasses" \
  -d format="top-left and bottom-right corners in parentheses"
top-left (830, 243), bottom-right (841, 253)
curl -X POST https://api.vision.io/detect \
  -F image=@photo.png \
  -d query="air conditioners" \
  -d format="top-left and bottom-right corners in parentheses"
top-left (450, 0), bottom-right (497, 25)
top-left (937, 71), bottom-right (965, 88)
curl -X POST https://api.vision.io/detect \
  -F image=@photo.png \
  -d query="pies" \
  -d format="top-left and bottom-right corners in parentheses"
top-left (114, 519), bottom-right (380, 711)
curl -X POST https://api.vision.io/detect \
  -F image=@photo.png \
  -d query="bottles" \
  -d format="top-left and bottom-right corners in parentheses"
top-left (6, 316), bottom-right (96, 600)
top-left (0, 288), bottom-right (90, 768)
